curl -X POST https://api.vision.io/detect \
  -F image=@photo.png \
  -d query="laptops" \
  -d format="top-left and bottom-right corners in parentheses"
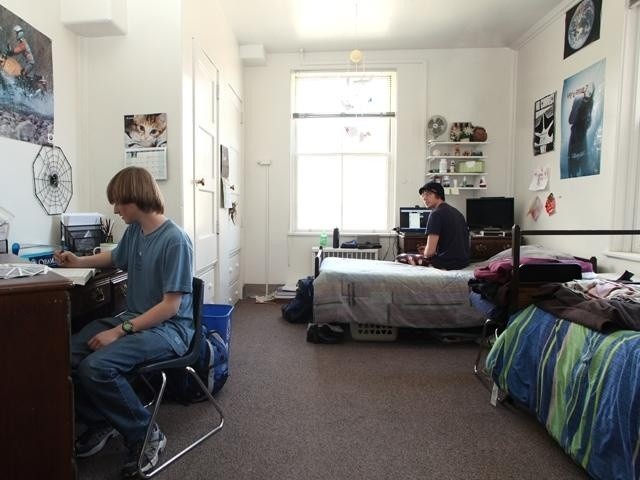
top-left (399, 207), bottom-right (432, 236)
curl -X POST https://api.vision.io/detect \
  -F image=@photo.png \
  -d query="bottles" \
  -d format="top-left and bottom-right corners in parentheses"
top-left (319, 229), bottom-right (327, 248)
top-left (463, 175), bottom-right (467, 186)
top-left (333, 228), bottom-right (339, 247)
top-left (439, 159), bottom-right (447, 173)
top-left (455, 149), bottom-right (460, 156)
top-left (443, 176), bottom-right (450, 187)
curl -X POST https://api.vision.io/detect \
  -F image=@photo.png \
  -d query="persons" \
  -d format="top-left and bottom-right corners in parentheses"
top-left (568, 82), bottom-right (594, 178)
top-left (53, 166), bottom-right (196, 479)
top-left (8, 25), bottom-right (35, 80)
top-left (416, 182), bottom-right (469, 270)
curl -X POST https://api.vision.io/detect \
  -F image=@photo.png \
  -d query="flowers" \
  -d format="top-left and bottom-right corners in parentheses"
top-left (453, 127), bottom-right (474, 140)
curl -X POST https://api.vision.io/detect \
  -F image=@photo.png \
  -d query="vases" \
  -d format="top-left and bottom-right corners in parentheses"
top-left (460, 137), bottom-right (470, 141)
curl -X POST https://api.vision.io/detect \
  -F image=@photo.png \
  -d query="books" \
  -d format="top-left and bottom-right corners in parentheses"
top-left (50, 268), bottom-right (101, 286)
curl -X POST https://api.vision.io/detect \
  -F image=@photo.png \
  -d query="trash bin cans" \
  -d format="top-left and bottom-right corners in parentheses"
top-left (200, 303), bottom-right (235, 356)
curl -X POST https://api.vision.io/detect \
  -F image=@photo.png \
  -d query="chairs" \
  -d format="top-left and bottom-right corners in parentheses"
top-left (130, 277), bottom-right (227, 479)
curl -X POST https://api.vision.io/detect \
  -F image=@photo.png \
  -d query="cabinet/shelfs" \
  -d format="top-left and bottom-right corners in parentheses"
top-left (426, 141), bottom-right (489, 189)
top-left (224, 82), bottom-right (242, 308)
top-left (190, 35), bottom-right (220, 307)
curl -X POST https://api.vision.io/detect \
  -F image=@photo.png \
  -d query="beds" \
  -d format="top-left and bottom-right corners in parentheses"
top-left (505, 223), bottom-right (640, 479)
top-left (312, 244), bottom-right (599, 336)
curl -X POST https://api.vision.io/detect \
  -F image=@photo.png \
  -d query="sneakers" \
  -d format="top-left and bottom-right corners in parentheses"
top-left (121, 421), bottom-right (167, 479)
top-left (74, 423), bottom-right (121, 458)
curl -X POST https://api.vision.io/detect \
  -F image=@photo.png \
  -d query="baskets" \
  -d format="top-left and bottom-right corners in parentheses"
top-left (350, 317), bottom-right (397, 341)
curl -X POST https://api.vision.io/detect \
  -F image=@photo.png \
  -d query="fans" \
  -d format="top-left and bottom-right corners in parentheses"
top-left (426, 115), bottom-right (448, 141)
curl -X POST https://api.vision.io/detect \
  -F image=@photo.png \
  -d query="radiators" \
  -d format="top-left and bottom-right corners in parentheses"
top-left (312, 247), bottom-right (379, 263)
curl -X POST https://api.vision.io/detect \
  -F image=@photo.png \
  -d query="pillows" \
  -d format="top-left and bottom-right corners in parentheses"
top-left (488, 246), bottom-right (576, 261)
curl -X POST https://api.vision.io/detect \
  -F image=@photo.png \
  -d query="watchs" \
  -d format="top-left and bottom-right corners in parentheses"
top-left (122, 320), bottom-right (134, 335)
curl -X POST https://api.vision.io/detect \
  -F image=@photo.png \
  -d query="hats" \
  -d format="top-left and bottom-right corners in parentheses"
top-left (418, 182), bottom-right (445, 199)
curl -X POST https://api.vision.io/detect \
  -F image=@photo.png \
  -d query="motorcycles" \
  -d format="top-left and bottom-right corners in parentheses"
top-left (0, 42), bottom-right (48, 106)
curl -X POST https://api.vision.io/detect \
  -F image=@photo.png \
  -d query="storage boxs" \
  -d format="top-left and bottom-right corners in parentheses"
top-left (202, 303), bottom-right (235, 344)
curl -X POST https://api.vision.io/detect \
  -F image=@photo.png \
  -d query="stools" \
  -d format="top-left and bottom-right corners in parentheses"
top-left (473, 314), bottom-right (508, 403)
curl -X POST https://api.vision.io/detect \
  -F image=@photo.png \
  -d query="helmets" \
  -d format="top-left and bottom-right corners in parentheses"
top-left (14, 25), bottom-right (23, 39)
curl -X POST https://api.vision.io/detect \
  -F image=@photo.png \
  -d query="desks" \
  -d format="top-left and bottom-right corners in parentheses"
top-left (397, 233), bottom-right (523, 262)
top-left (0, 253), bottom-right (130, 480)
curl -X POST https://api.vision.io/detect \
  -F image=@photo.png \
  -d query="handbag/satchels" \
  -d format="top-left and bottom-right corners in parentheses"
top-left (281, 278), bottom-right (314, 324)
top-left (193, 331), bottom-right (229, 404)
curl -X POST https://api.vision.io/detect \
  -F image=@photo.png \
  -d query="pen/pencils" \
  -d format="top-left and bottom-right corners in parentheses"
top-left (100, 217), bottom-right (115, 243)
top-left (61, 240), bottom-right (65, 264)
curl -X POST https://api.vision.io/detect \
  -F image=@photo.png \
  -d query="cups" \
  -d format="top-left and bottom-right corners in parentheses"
top-left (93, 242), bottom-right (119, 255)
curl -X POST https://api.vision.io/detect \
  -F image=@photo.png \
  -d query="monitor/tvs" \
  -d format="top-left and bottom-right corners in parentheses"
top-left (466, 196), bottom-right (514, 233)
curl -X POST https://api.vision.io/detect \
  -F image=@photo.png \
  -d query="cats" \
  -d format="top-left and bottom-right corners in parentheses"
top-left (124, 113), bottom-right (166, 150)
